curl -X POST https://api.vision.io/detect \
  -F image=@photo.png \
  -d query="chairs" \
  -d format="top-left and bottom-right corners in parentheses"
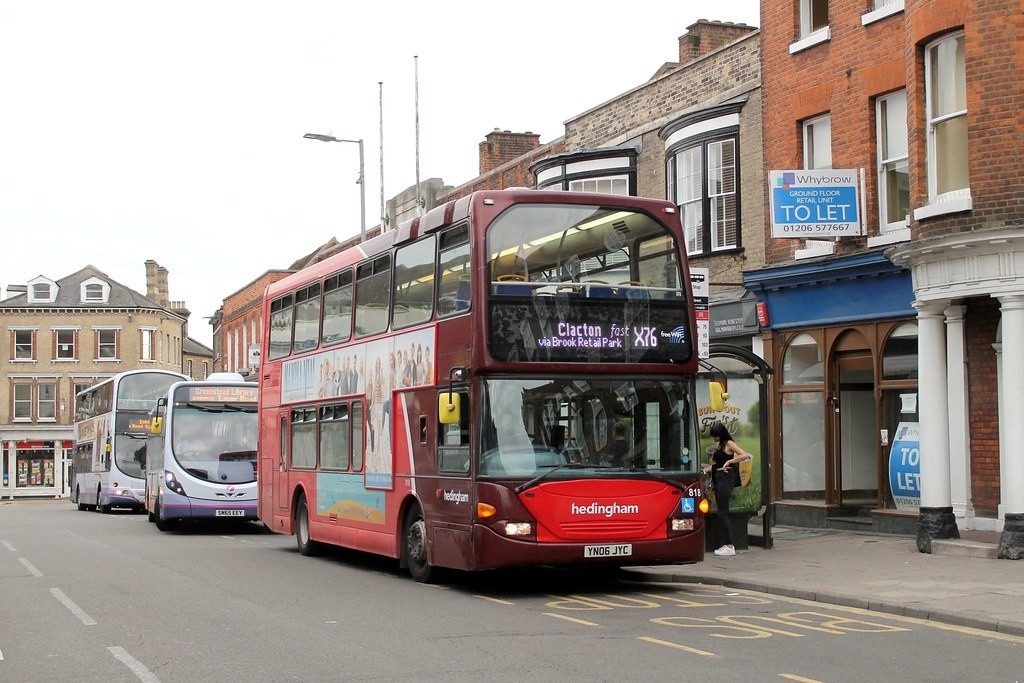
top-left (580, 280), bottom-right (614, 298)
top-left (555, 286), bottom-right (582, 298)
top-left (617, 281), bottom-right (651, 298)
top-left (455, 274), bottom-right (471, 311)
top-left (385, 301), bottom-right (409, 329)
top-left (361, 303), bottom-right (387, 334)
top-left (496, 275), bottom-right (532, 295)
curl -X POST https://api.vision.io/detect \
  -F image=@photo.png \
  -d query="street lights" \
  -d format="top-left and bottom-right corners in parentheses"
top-left (304, 133), bottom-right (367, 244)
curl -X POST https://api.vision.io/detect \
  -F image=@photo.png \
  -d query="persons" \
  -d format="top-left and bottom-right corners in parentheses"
top-left (603, 421), bottom-right (630, 468)
top-left (319, 342), bottom-right (433, 472)
top-left (702, 422), bottom-right (748, 555)
top-left (175, 429), bottom-right (208, 459)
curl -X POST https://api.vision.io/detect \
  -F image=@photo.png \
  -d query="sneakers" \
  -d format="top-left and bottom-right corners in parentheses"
top-left (714, 545), bottom-right (736, 556)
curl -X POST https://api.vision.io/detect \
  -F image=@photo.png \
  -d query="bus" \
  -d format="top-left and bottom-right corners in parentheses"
top-left (144, 372), bottom-right (271, 533)
top-left (72, 369), bottom-right (195, 514)
top-left (255, 186), bottom-right (730, 589)
